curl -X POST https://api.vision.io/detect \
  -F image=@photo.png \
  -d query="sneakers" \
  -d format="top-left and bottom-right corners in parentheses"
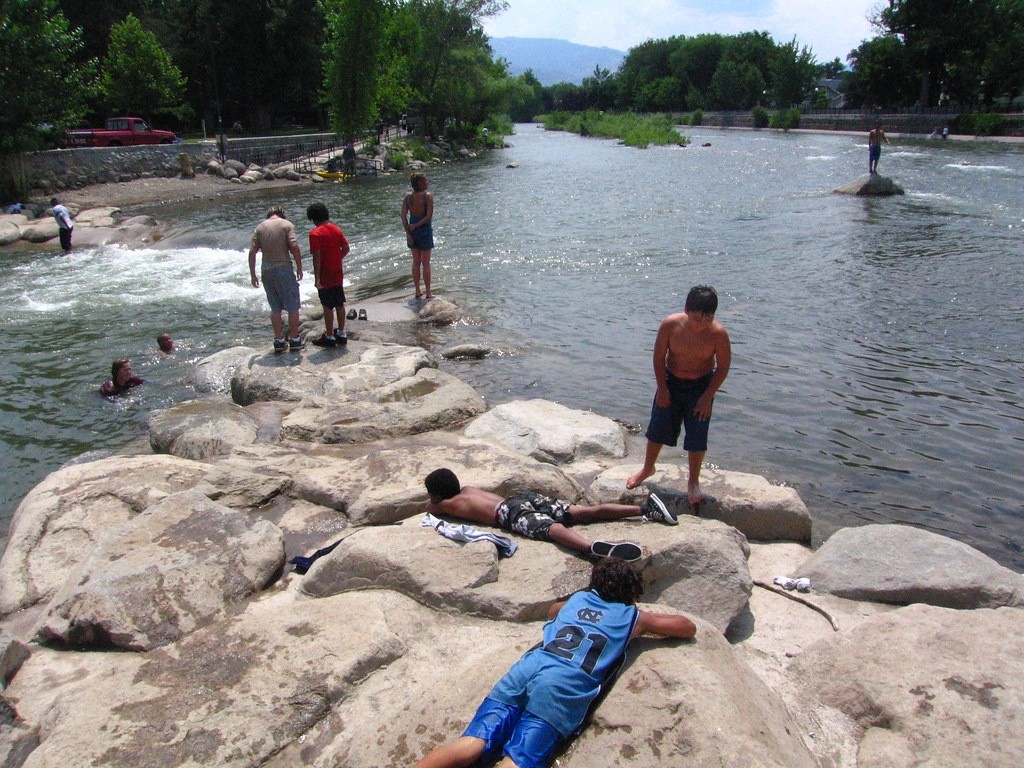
top-left (289, 335), bottom-right (306, 350)
top-left (591, 540), bottom-right (641, 563)
top-left (273, 336), bottom-right (287, 350)
top-left (640, 493), bottom-right (678, 525)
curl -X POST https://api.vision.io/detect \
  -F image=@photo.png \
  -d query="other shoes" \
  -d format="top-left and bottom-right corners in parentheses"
top-left (333, 327), bottom-right (348, 344)
top-left (347, 309), bottom-right (357, 320)
top-left (359, 309), bottom-right (367, 320)
top-left (311, 332), bottom-right (336, 346)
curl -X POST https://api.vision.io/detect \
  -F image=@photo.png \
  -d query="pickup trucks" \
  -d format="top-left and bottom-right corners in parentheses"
top-left (63, 117), bottom-right (176, 147)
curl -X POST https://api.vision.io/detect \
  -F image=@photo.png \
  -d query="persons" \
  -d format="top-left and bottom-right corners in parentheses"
top-left (306, 203), bottom-right (351, 347)
top-left (136, 122), bottom-right (148, 131)
top-left (9, 199), bottom-right (25, 213)
top-left (425, 469), bottom-right (679, 562)
top-left (411, 556), bottom-right (696, 768)
top-left (248, 206), bottom-right (305, 352)
top-left (156, 333), bottom-right (182, 355)
top-left (101, 359), bottom-right (145, 397)
top-left (232, 120), bottom-right (243, 133)
top-left (50, 196), bottom-right (74, 254)
top-left (401, 173), bottom-right (435, 298)
top-left (626, 284), bottom-right (731, 504)
top-left (867, 122), bottom-right (889, 173)
top-left (482, 126), bottom-right (489, 141)
top-left (931, 125), bottom-right (948, 139)
